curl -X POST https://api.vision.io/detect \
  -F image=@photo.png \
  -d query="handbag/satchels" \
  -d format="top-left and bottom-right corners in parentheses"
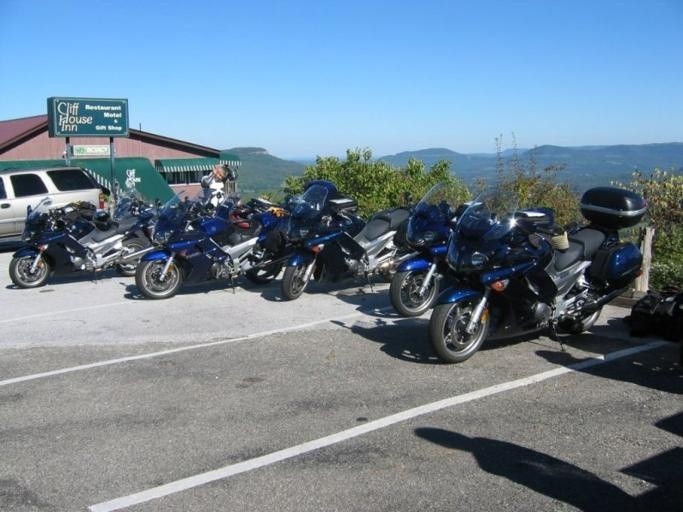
top-left (629, 283), bottom-right (683, 339)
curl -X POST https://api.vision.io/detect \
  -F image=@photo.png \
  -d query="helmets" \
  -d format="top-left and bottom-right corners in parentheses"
top-left (226, 165), bottom-right (239, 181)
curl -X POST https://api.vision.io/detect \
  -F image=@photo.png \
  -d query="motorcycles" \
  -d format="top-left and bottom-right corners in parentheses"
top-left (428, 186), bottom-right (650, 365)
top-left (8, 187), bottom-right (161, 289)
top-left (280, 183), bottom-right (417, 301)
top-left (388, 181), bottom-right (556, 318)
top-left (135, 180), bottom-right (339, 300)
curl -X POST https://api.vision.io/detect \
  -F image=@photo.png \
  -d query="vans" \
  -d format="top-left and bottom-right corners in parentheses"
top-left (0, 166), bottom-right (117, 239)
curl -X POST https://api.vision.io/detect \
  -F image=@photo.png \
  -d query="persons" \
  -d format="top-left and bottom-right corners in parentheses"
top-left (200, 163), bottom-right (237, 208)
